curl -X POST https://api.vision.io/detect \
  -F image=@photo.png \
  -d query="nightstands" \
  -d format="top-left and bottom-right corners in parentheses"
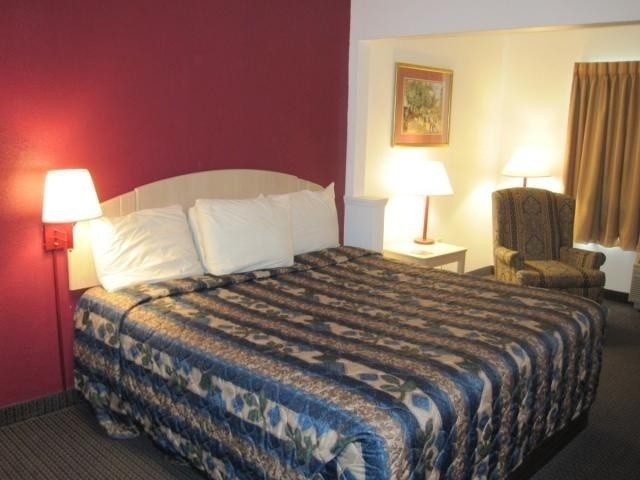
top-left (383, 240), bottom-right (468, 274)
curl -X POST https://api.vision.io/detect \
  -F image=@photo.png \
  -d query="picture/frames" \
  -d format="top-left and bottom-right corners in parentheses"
top-left (390, 60), bottom-right (453, 146)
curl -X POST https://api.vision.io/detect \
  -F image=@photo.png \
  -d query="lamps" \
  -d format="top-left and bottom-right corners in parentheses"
top-left (500, 144), bottom-right (553, 186)
top-left (395, 160), bottom-right (454, 244)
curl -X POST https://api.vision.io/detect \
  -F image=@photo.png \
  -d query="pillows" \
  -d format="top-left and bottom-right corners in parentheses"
top-left (42, 168), bottom-right (102, 251)
top-left (188, 198), bottom-right (294, 277)
top-left (260, 181), bottom-right (339, 257)
top-left (92, 206), bottom-right (203, 292)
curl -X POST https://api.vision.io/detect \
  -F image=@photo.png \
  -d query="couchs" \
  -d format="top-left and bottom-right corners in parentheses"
top-left (492, 187), bottom-right (605, 307)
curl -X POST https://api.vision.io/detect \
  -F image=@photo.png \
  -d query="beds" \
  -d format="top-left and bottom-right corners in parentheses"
top-left (66, 168), bottom-right (608, 479)
